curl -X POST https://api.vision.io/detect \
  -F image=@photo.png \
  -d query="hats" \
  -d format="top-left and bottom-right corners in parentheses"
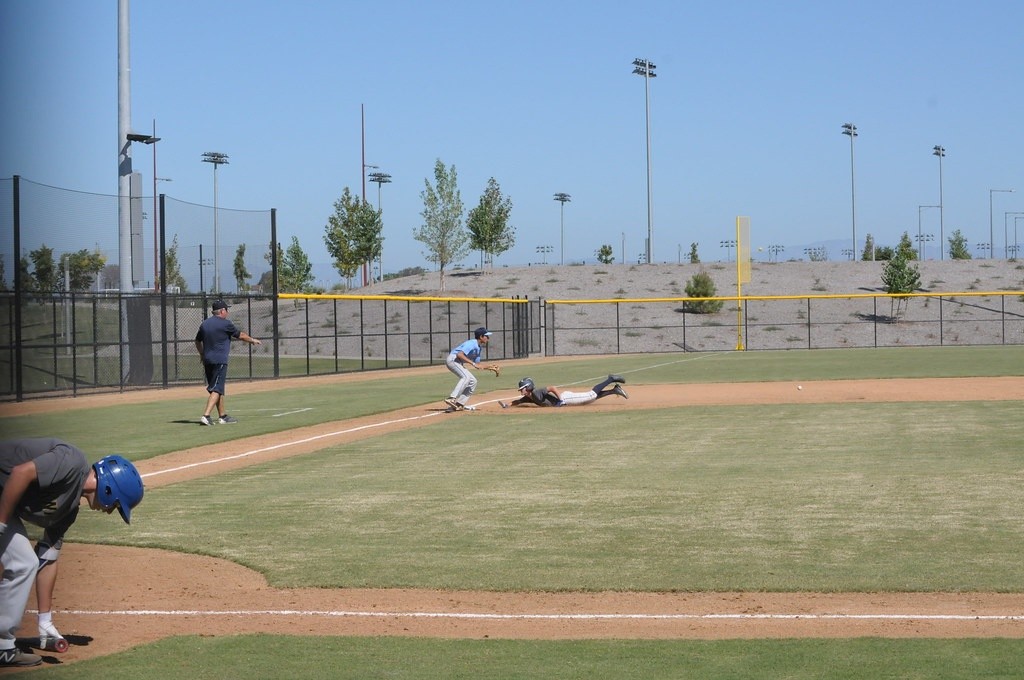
top-left (474, 328), bottom-right (493, 339)
top-left (212, 300), bottom-right (232, 311)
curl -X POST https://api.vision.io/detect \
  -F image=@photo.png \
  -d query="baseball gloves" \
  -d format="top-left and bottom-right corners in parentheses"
top-left (486, 364), bottom-right (500, 377)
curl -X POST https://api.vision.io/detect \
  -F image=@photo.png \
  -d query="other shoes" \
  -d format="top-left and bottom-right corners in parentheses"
top-left (219, 415), bottom-right (238, 425)
top-left (200, 416), bottom-right (216, 426)
top-left (445, 397), bottom-right (464, 412)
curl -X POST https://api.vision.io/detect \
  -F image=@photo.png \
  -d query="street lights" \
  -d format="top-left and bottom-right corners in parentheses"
top-left (535, 245), bottom-right (555, 266)
top-left (201, 151), bottom-right (231, 294)
top-left (553, 192), bottom-right (572, 266)
top-left (719, 240), bottom-right (737, 262)
top-left (915, 233), bottom-right (935, 261)
top-left (918, 205), bottom-right (943, 261)
top-left (632, 57), bottom-right (657, 265)
top-left (841, 122), bottom-right (858, 260)
top-left (977, 243), bottom-right (990, 259)
top-left (989, 189), bottom-right (1017, 258)
top-left (768, 244), bottom-right (784, 263)
top-left (367, 172), bottom-right (393, 283)
top-left (933, 144), bottom-right (945, 259)
top-left (1008, 245), bottom-right (1020, 258)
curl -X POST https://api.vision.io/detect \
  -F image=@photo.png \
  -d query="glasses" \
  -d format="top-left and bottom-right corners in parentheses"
top-left (486, 336), bottom-right (489, 339)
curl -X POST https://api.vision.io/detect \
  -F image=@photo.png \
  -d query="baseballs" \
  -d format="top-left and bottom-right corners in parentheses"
top-left (797, 385), bottom-right (802, 391)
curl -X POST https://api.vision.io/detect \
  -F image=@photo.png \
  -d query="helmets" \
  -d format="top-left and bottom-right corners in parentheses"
top-left (518, 377), bottom-right (534, 391)
top-left (91, 456), bottom-right (144, 526)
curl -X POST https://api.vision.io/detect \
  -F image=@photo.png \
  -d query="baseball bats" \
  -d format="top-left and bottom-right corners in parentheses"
top-left (15, 637), bottom-right (69, 653)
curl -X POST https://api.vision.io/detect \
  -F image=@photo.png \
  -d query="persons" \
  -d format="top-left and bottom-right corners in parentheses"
top-left (195, 300), bottom-right (262, 425)
top-left (444, 327), bottom-right (499, 412)
top-left (498, 374), bottom-right (629, 408)
top-left (0, 437), bottom-right (144, 666)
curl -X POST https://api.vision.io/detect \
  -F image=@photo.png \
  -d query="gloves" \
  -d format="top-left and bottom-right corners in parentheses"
top-left (498, 401), bottom-right (508, 408)
top-left (38, 610), bottom-right (65, 649)
top-left (556, 400), bottom-right (566, 408)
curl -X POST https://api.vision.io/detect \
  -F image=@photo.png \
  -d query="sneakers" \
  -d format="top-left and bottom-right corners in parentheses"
top-left (608, 374), bottom-right (625, 383)
top-left (614, 384), bottom-right (628, 400)
top-left (0, 648), bottom-right (43, 666)
